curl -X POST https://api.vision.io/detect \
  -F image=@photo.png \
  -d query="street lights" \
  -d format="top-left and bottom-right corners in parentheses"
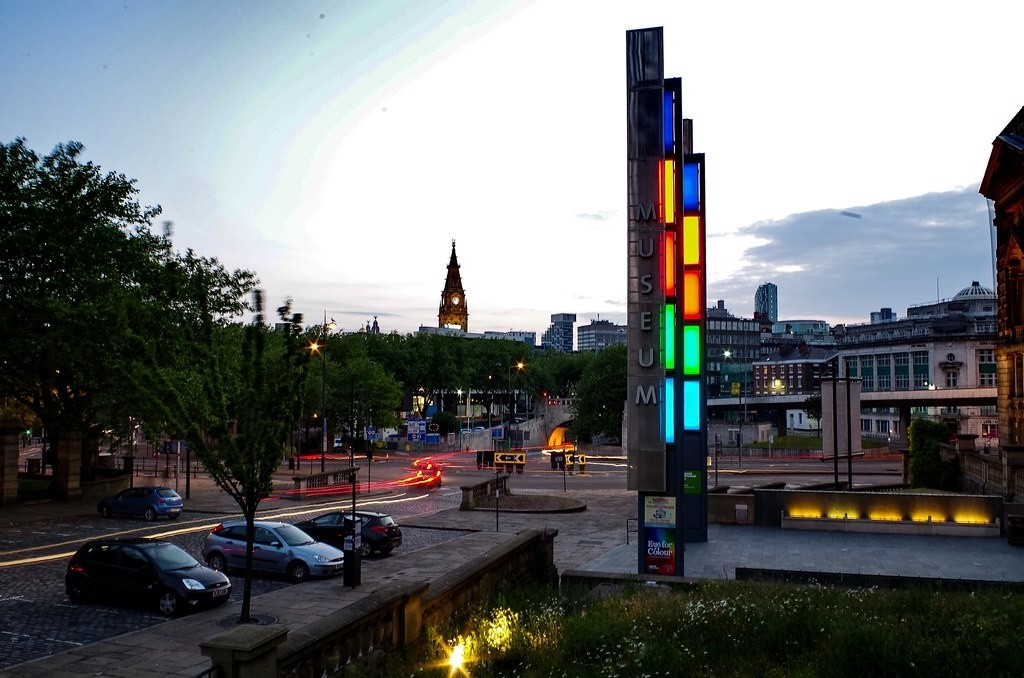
top-left (345, 438), bottom-right (361, 560)
top-left (507, 363), bottom-right (523, 453)
top-left (724, 349), bottom-right (745, 468)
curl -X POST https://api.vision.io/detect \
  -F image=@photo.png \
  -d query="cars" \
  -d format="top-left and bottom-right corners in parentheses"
top-left (201, 520), bottom-right (346, 585)
top-left (97, 486), bottom-right (184, 521)
top-left (292, 507), bottom-right (402, 558)
top-left (333, 438), bottom-right (342, 450)
top-left (64, 538), bottom-right (230, 618)
